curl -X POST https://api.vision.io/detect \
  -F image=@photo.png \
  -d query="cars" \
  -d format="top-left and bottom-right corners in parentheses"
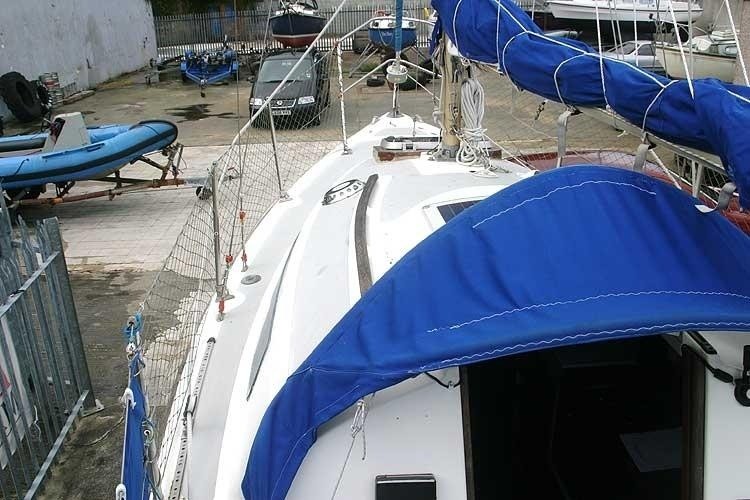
top-left (600, 39), bottom-right (676, 76)
top-left (246, 48), bottom-right (330, 128)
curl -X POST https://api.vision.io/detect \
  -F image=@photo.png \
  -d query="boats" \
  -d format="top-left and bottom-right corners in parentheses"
top-left (268, 0), bottom-right (329, 48)
top-left (649, 0), bottom-right (739, 82)
top-left (366, 8), bottom-right (421, 53)
top-left (118, 0), bottom-right (750, 500)
top-left (0, 110), bottom-right (179, 190)
top-left (545, 0), bottom-right (705, 27)
